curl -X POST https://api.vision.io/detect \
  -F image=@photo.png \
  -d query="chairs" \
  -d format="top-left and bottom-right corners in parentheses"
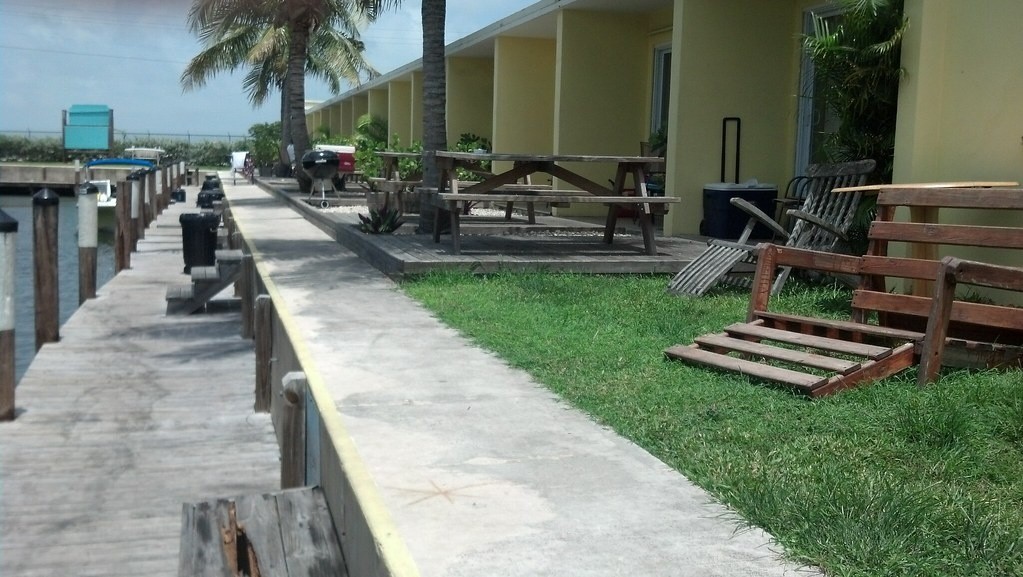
top-left (668, 161), bottom-right (875, 296)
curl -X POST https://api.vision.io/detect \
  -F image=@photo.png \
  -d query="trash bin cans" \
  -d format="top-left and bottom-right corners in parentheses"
top-left (204, 173), bottom-right (218, 180)
top-left (179, 214), bottom-right (221, 266)
top-left (703, 183), bottom-right (776, 238)
top-left (198, 191), bottom-right (225, 208)
top-left (202, 179), bottom-right (221, 190)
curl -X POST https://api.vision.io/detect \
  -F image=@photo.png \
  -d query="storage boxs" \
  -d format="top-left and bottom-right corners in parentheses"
top-left (700, 184), bottom-right (777, 243)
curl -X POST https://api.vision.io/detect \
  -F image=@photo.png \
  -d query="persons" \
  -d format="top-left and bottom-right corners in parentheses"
top-left (244, 154), bottom-right (252, 179)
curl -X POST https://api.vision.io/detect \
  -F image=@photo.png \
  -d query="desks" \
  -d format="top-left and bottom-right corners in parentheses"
top-left (373, 151), bottom-right (420, 217)
top-left (830, 182), bottom-right (1020, 299)
top-left (432, 150), bottom-right (665, 257)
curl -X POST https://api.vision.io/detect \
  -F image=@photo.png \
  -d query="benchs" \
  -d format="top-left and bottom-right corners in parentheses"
top-left (368, 177), bottom-right (682, 206)
top-left (664, 187), bottom-right (1022, 394)
